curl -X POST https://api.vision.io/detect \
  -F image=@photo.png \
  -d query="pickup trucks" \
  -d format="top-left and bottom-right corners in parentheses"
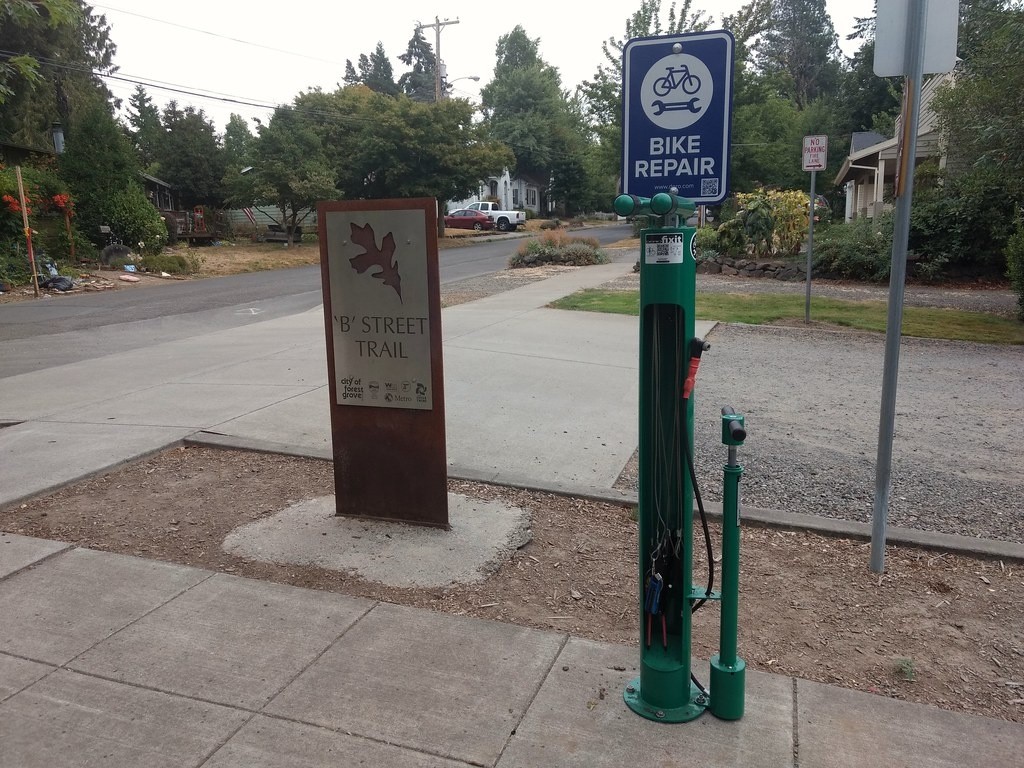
top-left (448, 201), bottom-right (526, 232)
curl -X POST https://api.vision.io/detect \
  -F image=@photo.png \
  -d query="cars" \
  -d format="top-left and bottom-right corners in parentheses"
top-left (436, 208), bottom-right (496, 232)
